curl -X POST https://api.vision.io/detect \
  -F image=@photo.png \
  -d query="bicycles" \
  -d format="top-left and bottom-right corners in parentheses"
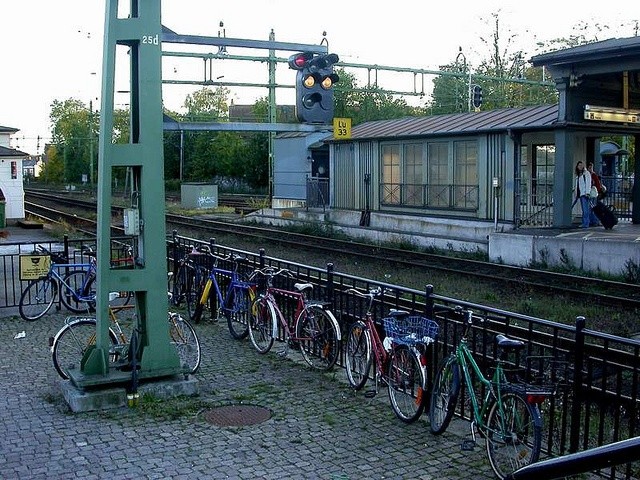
top-left (246, 266), bottom-right (341, 373)
top-left (48, 292), bottom-right (202, 380)
top-left (19, 244), bottom-right (131, 322)
top-left (195, 244), bottom-right (258, 339)
top-left (60, 239), bottom-right (138, 312)
top-left (430, 302), bottom-right (555, 480)
top-left (173, 236), bottom-right (203, 320)
top-left (344, 286), bottom-right (440, 425)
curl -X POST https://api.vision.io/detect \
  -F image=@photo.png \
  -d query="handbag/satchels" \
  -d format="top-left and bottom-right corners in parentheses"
top-left (592, 172), bottom-right (608, 200)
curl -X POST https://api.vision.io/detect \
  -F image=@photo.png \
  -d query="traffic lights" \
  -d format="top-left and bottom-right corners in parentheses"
top-left (296, 52), bottom-right (339, 124)
top-left (288, 51), bottom-right (327, 74)
top-left (472, 85), bottom-right (483, 109)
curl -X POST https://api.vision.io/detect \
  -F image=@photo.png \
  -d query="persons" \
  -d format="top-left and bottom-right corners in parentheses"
top-left (575, 161), bottom-right (591, 228)
top-left (587, 162), bottom-right (602, 226)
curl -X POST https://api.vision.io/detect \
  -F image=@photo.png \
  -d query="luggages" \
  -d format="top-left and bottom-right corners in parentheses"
top-left (589, 202), bottom-right (618, 230)
